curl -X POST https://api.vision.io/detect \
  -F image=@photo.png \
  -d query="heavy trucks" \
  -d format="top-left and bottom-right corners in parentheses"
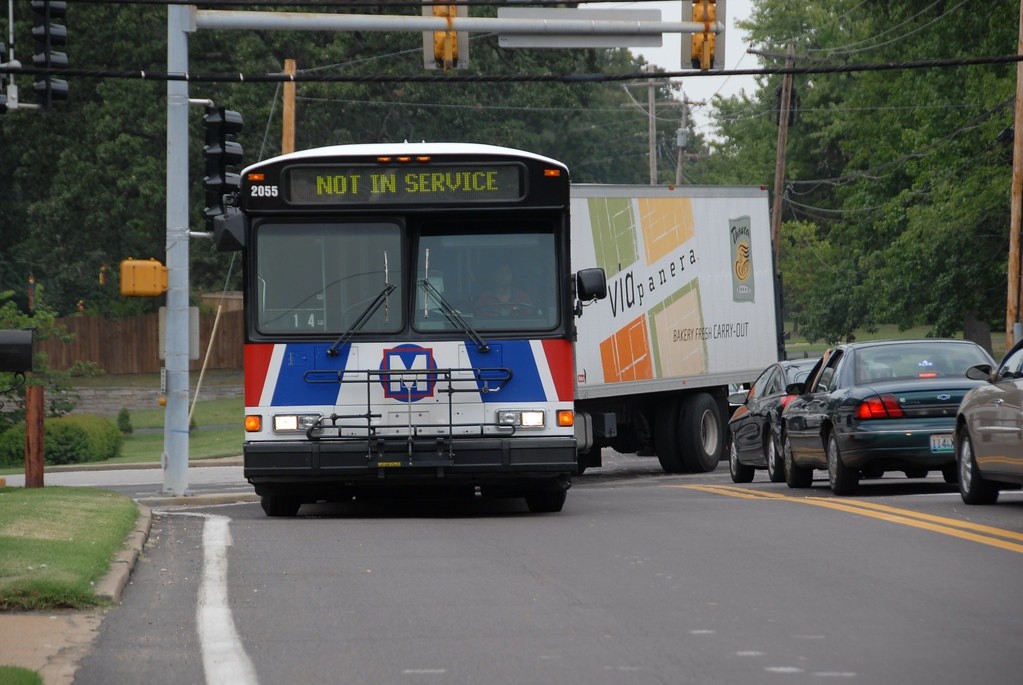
top-left (213, 139), bottom-right (780, 517)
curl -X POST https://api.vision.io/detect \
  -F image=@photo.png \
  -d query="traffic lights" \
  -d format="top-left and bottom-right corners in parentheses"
top-left (202, 105), bottom-right (245, 234)
top-left (30, 0), bottom-right (69, 106)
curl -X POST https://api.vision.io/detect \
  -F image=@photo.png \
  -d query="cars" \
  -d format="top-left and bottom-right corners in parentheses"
top-left (780, 339), bottom-right (999, 496)
top-left (726, 358), bottom-right (830, 484)
top-left (953, 335), bottom-right (1023, 505)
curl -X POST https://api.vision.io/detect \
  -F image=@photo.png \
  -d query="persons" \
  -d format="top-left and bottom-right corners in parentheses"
top-left (476, 263), bottom-right (531, 317)
top-left (811, 348), bottom-right (831, 392)
